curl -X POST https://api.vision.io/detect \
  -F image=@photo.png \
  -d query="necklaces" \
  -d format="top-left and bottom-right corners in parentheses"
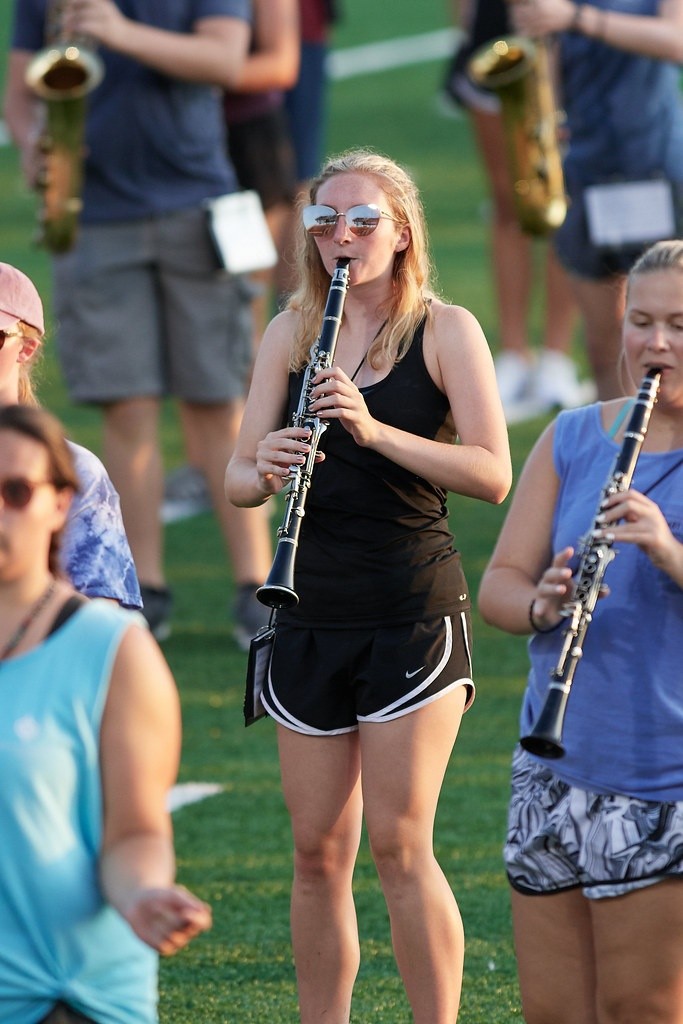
top-left (0, 581), bottom-right (59, 662)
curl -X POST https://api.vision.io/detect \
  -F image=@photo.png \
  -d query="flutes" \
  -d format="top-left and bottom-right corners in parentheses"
top-left (255, 256), bottom-right (352, 609)
top-left (518, 364), bottom-right (663, 756)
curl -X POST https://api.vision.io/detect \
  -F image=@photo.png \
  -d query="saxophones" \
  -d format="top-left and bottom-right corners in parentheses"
top-left (466, 3), bottom-right (572, 230)
top-left (28, 0), bottom-right (107, 250)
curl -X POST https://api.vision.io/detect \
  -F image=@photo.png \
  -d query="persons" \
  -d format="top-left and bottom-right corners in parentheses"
top-left (0, 261), bottom-right (144, 611)
top-left (223, 153), bottom-right (512, 1024)
top-left (8, 0), bottom-right (340, 651)
top-left (0, 403), bottom-right (213, 1024)
top-left (439, 0), bottom-right (683, 428)
top-left (476, 239), bottom-right (682, 1024)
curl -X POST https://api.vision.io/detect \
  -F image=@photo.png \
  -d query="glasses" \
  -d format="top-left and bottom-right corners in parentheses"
top-left (302, 205), bottom-right (404, 236)
top-left (0, 475), bottom-right (55, 508)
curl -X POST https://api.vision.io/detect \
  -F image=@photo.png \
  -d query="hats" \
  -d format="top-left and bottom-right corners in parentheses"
top-left (0, 263), bottom-right (44, 332)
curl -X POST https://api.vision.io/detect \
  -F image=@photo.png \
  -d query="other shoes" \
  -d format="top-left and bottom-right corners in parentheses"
top-left (494, 351), bottom-right (527, 405)
top-left (235, 588), bottom-right (275, 648)
top-left (135, 586), bottom-right (169, 632)
top-left (499, 357), bottom-right (591, 421)
